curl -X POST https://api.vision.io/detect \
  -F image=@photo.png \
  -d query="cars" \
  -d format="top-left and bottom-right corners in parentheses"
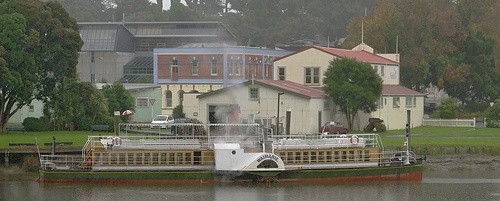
top-left (151, 114), bottom-right (175, 130)
top-left (321, 120), bottom-right (349, 135)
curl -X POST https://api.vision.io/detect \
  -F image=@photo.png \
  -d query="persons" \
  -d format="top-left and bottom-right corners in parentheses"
top-left (85, 157), bottom-right (92, 171)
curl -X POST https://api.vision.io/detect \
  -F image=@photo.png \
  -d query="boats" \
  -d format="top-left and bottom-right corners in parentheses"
top-left (37, 121), bottom-right (426, 186)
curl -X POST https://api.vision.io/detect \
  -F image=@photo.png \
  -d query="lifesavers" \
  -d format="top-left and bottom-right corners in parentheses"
top-left (350, 135), bottom-right (359, 145)
top-left (112, 137), bottom-right (122, 146)
